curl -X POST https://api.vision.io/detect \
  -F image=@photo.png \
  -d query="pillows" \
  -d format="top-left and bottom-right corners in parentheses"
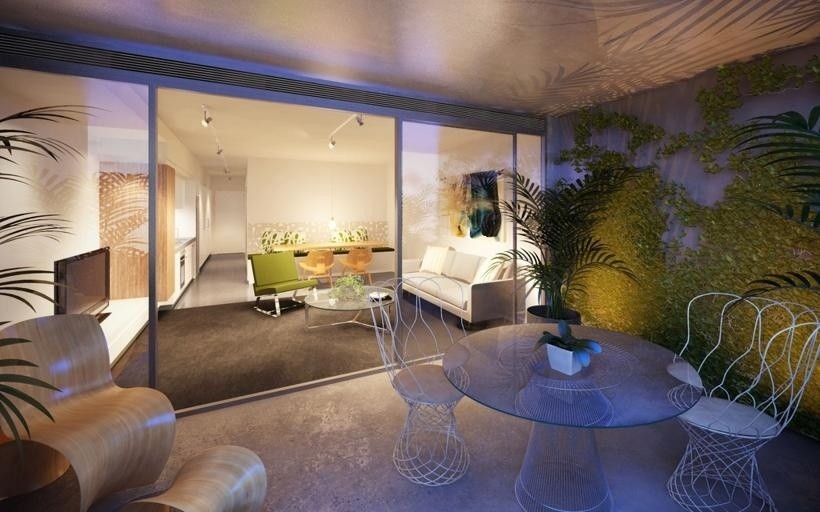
top-left (470, 257), bottom-right (506, 288)
top-left (419, 245), bottom-right (456, 276)
top-left (441, 249), bottom-right (480, 283)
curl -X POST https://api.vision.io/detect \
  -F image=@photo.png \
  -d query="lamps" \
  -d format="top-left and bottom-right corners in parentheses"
top-left (199, 104), bottom-right (232, 183)
top-left (328, 113), bottom-right (364, 149)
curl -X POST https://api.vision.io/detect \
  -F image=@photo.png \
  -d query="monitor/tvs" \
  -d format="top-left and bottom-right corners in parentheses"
top-left (54, 247), bottom-right (112, 325)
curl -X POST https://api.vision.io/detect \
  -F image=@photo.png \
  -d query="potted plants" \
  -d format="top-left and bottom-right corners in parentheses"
top-left (533, 318), bottom-right (602, 377)
top-left (483, 166), bottom-right (646, 326)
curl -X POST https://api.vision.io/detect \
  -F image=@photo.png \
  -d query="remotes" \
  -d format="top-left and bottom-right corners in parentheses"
top-left (370, 295), bottom-right (393, 302)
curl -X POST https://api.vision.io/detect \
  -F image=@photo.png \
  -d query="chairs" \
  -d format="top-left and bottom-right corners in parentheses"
top-left (338, 246), bottom-right (375, 285)
top-left (249, 251), bottom-right (318, 318)
top-left (1, 312), bottom-right (176, 512)
top-left (299, 248), bottom-right (335, 289)
top-left (366, 276), bottom-right (471, 487)
top-left (665, 291), bottom-right (820, 512)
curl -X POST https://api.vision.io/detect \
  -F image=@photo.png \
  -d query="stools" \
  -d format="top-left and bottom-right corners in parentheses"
top-left (118, 444), bottom-right (267, 512)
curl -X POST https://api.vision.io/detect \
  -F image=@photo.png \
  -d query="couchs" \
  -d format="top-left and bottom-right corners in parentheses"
top-left (402, 255), bottom-right (526, 330)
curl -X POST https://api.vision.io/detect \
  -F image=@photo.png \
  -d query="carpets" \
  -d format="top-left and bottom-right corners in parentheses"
top-left (110, 285), bottom-right (524, 411)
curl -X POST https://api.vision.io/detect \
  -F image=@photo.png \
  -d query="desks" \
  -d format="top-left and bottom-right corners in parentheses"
top-left (304, 286), bottom-right (395, 336)
top-left (442, 323), bottom-right (704, 512)
